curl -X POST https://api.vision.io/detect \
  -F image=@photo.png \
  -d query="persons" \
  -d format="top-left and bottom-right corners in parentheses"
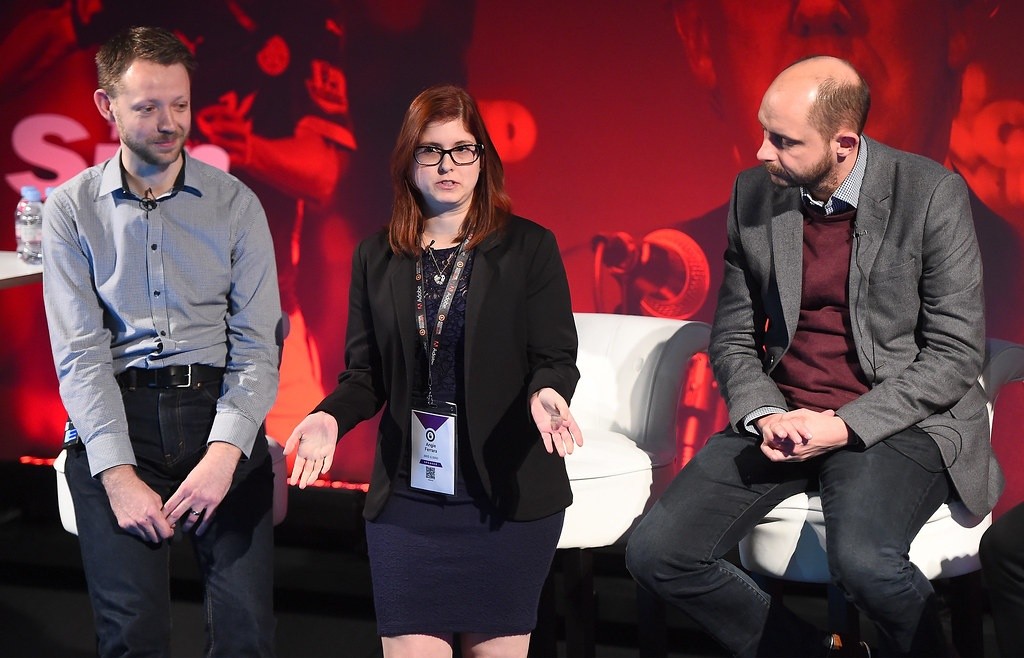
top-left (979, 501), bottom-right (1024, 658)
top-left (1, 1), bottom-right (1024, 507)
top-left (283, 86), bottom-right (584, 658)
top-left (625, 55), bottom-right (1006, 658)
top-left (41, 26), bottom-right (283, 658)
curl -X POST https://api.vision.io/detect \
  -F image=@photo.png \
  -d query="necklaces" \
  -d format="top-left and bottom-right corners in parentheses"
top-left (422, 224), bottom-right (469, 278)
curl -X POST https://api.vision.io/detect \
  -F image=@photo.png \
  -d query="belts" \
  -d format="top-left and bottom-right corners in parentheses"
top-left (116, 364), bottom-right (226, 388)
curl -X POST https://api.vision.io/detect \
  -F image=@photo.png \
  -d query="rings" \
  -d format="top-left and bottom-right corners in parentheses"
top-left (190, 509), bottom-right (201, 516)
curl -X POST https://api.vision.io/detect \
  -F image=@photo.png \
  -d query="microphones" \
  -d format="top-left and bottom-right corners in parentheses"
top-left (425, 240), bottom-right (435, 255)
top-left (852, 222), bottom-right (868, 238)
top-left (150, 200), bottom-right (157, 210)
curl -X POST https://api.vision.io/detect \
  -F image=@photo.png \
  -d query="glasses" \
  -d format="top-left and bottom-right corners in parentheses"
top-left (413, 144), bottom-right (484, 166)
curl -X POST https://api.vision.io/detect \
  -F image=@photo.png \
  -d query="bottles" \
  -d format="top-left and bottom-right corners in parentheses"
top-left (44, 187), bottom-right (54, 200)
top-left (14, 186), bottom-right (35, 255)
top-left (21, 191), bottom-right (43, 265)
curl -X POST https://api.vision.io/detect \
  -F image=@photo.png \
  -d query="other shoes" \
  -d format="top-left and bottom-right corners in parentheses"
top-left (857, 640), bottom-right (874, 658)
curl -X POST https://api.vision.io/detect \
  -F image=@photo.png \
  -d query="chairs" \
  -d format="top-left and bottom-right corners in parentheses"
top-left (533, 313), bottom-right (711, 658)
top-left (735, 321), bottom-right (1024, 658)
